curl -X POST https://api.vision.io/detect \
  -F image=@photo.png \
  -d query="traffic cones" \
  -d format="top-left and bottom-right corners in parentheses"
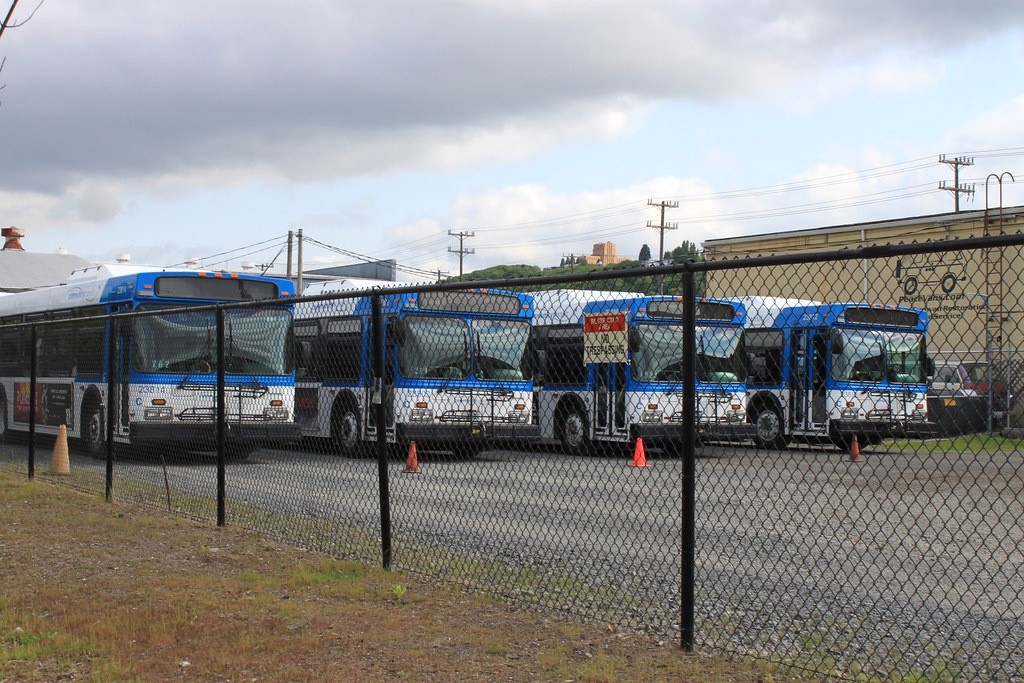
top-left (844, 435), bottom-right (865, 462)
top-left (628, 437), bottom-right (652, 468)
top-left (401, 441), bottom-right (420, 473)
top-left (41, 424), bottom-right (74, 476)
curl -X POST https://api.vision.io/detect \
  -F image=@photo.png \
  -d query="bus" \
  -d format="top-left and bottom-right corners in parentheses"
top-left (0, 248), bottom-right (303, 462)
top-left (292, 278), bottom-right (542, 461)
top-left (888, 359), bottom-right (1016, 411)
top-left (716, 296), bottom-right (939, 450)
top-left (519, 287), bottom-right (760, 457)
